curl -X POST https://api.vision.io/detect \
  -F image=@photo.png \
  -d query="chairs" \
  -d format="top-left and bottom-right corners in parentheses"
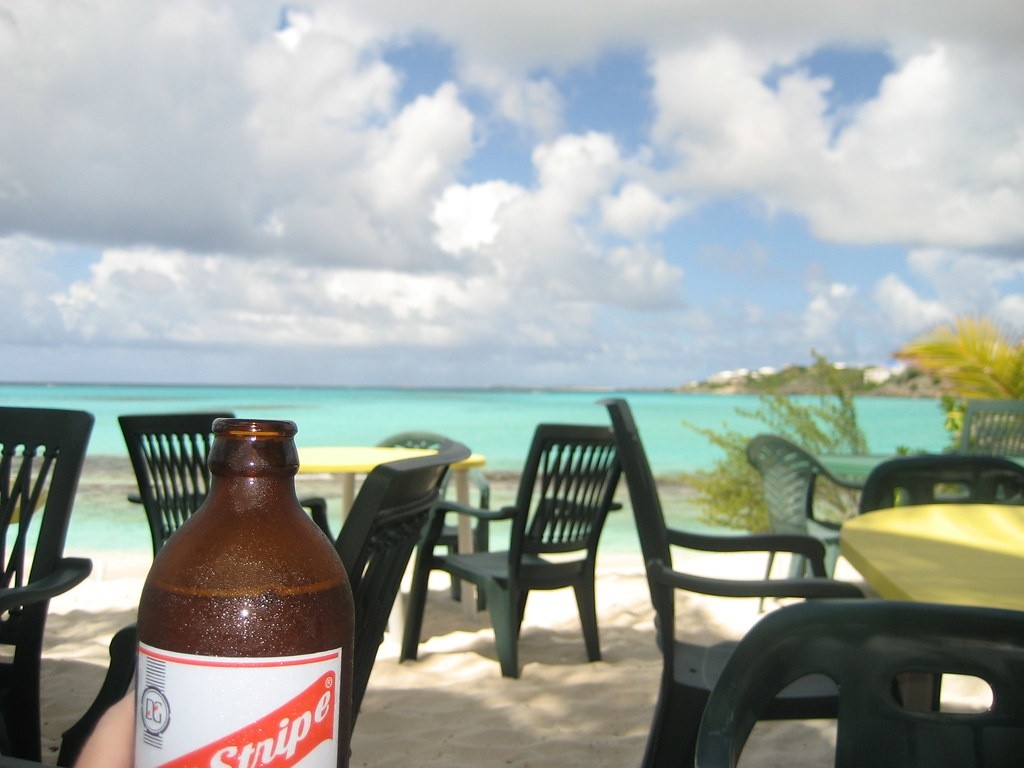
top-left (117, 410), bottom-right (326, 554)
top-left (0, 407), bottom-right (96, 768)
top-left (696, 599), bottom-right (1024, 768)
top-left (746, 434), bottom-right (863, 612)
top-left (958, 397), bottom-right (1024, 455)
top-left (377, 431), bottom-right (491, 612)
top-left (55, 442), bottom-right (471, 768)
top-left (595, 396), bottom-right (866, 768)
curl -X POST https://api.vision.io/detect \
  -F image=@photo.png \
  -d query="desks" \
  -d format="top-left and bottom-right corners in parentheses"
top-left (838, 504), bottom-right (1024, 611)
top-left (815, 456), bottom-right (1024, 480)
top-left (400, 423), bottom-right (623, 678)
top-left (296, 447), bottom-right (485, 645)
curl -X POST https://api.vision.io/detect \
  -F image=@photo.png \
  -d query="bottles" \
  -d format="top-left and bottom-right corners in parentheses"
top-left (133, 419), bottom-right (356, 767)
